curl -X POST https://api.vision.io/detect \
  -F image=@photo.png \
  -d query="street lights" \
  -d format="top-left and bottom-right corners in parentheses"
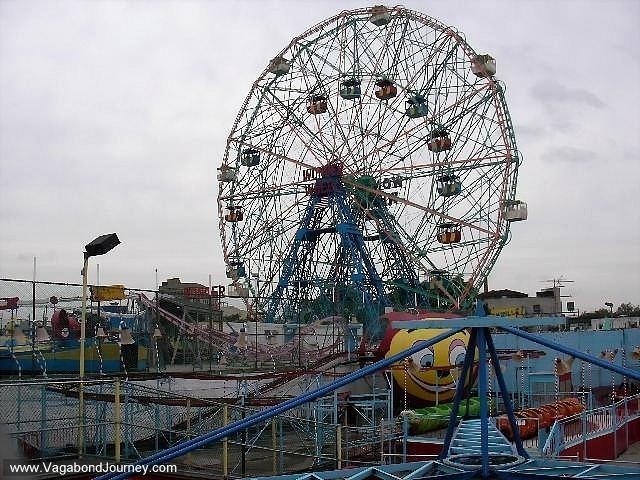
top-left (604, 301), bottom-right (615, 331)
top-left (74, 228), bottom-right (122, 459)
